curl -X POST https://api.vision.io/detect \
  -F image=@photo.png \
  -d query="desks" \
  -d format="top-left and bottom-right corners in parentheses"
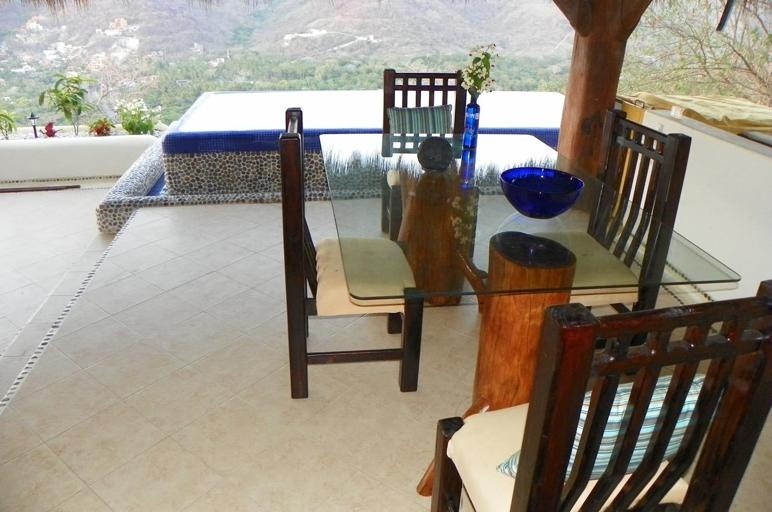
top-left (317, 134), bottom-right (742, 496)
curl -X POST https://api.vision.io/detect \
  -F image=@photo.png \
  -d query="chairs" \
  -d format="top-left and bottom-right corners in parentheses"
top-left (381, 68), bottom-right (467, 233)
top-left (430, 279), bottom-right (771, 512)
top-left (279, 108), bottom-right (423, 391)
top-left (587, 108), bottom-right (691, 312)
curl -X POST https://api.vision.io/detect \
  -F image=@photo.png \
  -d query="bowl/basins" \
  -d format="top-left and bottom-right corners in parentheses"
top-left (499, 167), bottom-right (585, 219)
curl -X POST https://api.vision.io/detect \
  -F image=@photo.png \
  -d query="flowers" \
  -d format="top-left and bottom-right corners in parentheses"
top-left (114, 98), bottom-right (162, 134)
top-left (459, 42), bottom-right (501, 98)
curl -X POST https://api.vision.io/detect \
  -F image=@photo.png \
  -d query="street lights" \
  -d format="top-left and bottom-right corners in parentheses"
top-left (28, 112), bottom-right (39, 138)
